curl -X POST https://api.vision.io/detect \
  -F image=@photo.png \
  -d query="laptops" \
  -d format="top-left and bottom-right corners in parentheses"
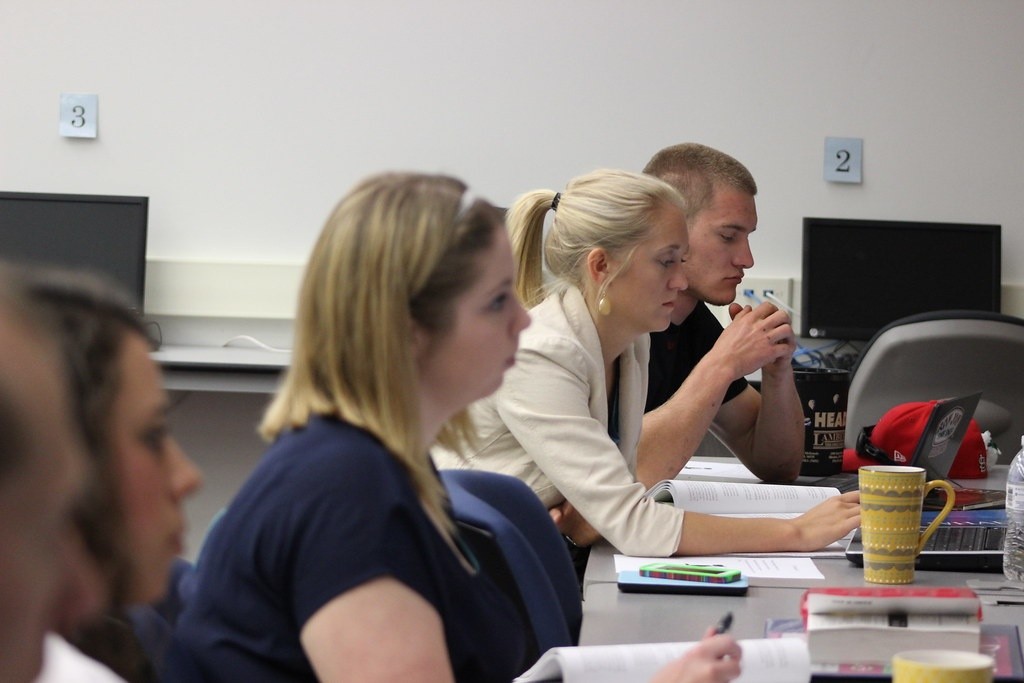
top-left (845, 524), bottom-right (1011, 572)
top-left (805, 389), bottom-right (983, 501)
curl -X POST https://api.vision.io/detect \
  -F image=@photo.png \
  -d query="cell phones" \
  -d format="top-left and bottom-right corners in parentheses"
top-left (641, 563), bottom-right (741, 583)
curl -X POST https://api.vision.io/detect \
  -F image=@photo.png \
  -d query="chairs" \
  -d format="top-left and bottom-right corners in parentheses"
top-left (438, 470), bottom-right (583, 662)
top-left (69, 557), bottom-right (194, 683)
top-left (844, 310), bottom-right (1024, 464)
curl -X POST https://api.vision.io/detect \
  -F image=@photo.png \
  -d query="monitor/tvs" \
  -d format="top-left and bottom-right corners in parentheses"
top-left (0, 191), bottom-right (149, 319)
top-left (801, 218), bottom-right (1002, 341)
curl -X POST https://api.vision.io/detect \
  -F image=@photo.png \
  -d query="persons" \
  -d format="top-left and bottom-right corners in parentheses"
top-left (170, 172), bottom-right (742, 683)
top-left (432, 173), bottom-right (864, 561)
top-left (0, 271), bottom-right (206, 683)
top-left (628, 143), bottom-right (811, 484)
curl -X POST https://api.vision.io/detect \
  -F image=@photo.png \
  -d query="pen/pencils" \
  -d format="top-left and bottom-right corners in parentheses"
top-left (709, 612), bottom-right (734, 638)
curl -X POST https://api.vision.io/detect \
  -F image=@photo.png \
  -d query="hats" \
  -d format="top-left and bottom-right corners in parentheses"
top-left (842, 400), bottom-right (987, 480)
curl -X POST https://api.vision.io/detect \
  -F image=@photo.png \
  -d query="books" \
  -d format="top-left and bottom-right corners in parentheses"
top-left (513, 638), bottom-right (812, 683)
top-left (640, 478), bottom-right (851, 559)
top-left (613, 547), bottom-right (825, 587)
top-left (804, 586), bottom-right (984, 666)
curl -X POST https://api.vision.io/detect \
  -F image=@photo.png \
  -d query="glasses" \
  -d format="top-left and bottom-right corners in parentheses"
top-left (857, 426), bottom-right (898, 465)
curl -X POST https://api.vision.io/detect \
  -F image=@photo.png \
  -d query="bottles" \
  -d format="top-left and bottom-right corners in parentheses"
top-left (1001, 434), bottom-right (1024, 580)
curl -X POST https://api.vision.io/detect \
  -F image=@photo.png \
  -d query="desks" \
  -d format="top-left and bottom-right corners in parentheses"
top-left (148, 344), bottom-right (763, 395)
top-left (582, 456), bottom-right (1024, 683)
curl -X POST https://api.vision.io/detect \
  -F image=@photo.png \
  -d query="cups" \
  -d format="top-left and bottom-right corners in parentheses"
top-left (893, 649), bottom-right (996, 683)
top-left (857, 465), bottom-right (955, 584)
